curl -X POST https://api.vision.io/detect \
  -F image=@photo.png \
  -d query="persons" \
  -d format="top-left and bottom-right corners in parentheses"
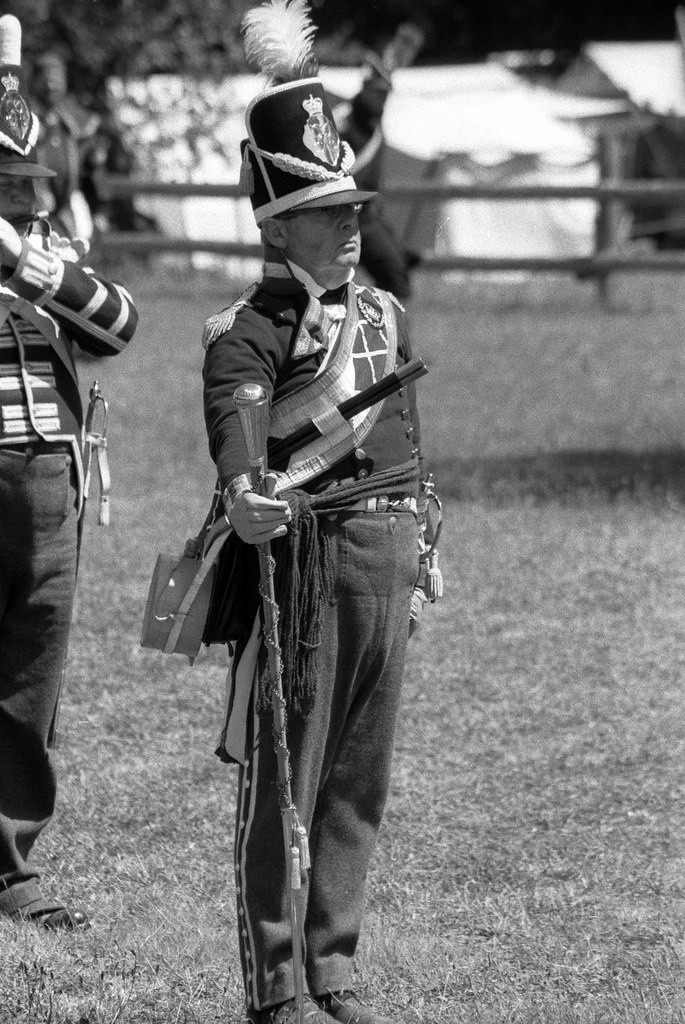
top-left (0, 110), bottom-right (139, 930)
top-left (22, 53), bottom-right (100, 254)
top-left (189, 78), bottom-right (442, 1022)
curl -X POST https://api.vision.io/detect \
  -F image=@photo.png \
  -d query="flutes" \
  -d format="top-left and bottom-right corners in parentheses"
top-left (267, 355), bottom-right (429, 470)
top-left (8, 210), bottom-right (49, 227)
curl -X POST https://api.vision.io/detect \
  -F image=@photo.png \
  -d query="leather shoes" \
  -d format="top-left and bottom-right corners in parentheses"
top-left (322, 993), bottom-right (393, 1024)
top-left (27, 908), bottom-right (93, 931)
top-left (246, 997), bottom-right (340, 1024)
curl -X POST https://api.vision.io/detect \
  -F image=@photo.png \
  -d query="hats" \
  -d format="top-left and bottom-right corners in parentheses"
top-left (240, 76), bottom-right (378, 226)
top-left (2, 14), bottom-right (54, 179)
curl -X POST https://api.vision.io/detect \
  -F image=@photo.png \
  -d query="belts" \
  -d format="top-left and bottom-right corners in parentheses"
top-left (14, 442), bottom-right (75, 455)
top-left (315, 493), bottom-right (421, 519)
